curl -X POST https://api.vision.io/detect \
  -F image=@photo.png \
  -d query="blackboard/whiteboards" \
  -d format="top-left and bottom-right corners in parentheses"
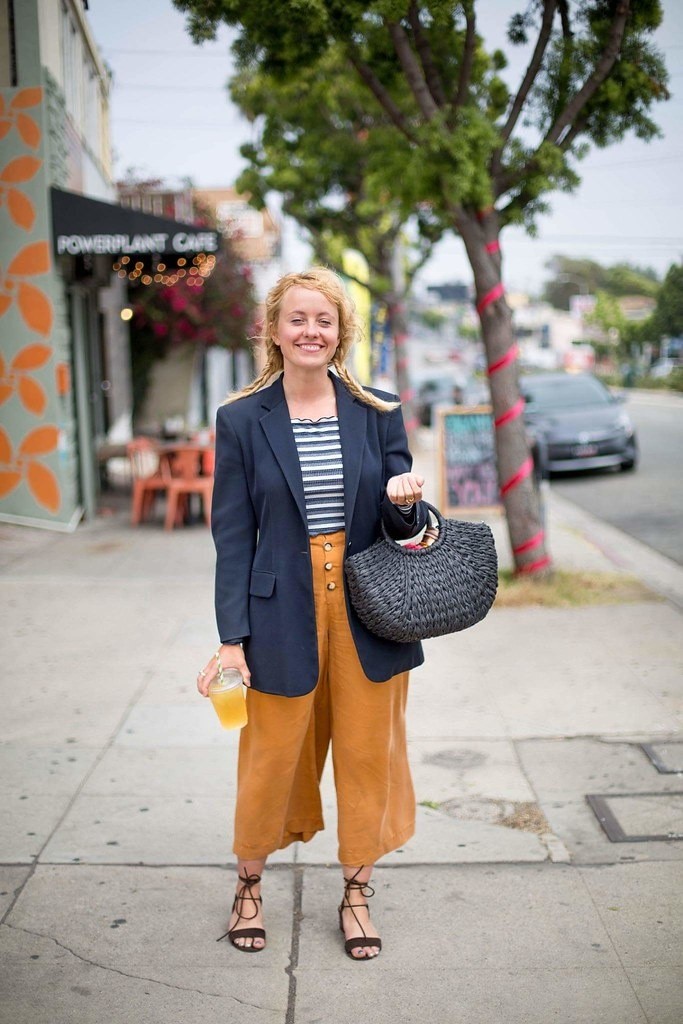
top-left (434, 403), bottom-right (508, 518)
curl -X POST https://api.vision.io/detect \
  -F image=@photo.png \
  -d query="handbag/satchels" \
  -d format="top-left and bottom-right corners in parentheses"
top-left (344, 500), bottom-right (498, 641)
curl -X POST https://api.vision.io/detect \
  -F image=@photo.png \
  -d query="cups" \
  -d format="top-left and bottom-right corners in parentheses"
top-left (208, 671), bottom-right (247, 730)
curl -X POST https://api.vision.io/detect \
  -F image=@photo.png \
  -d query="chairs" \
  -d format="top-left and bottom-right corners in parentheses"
top-left (126, 429), bottom-right (217, 531)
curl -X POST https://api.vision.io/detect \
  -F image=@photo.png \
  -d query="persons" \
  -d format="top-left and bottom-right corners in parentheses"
top-left (197, 265), bottom-right (431, 962)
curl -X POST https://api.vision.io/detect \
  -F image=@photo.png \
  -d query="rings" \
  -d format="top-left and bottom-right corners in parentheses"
top-left (406, 497), bottom-right (415, 504)
top-left (199, 669), bottom-right (207, 677)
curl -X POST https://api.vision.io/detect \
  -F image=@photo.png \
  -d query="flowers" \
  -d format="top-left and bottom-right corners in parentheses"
top-left (106, 178), bottom-right (268, 359)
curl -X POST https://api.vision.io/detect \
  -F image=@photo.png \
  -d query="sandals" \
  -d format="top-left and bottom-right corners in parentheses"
top-left (338, 864), bottom-right (381, 960)
top-left (216, 866), bottom-right (265, 952)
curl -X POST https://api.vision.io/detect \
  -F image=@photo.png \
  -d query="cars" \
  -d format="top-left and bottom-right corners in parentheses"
top-left (516, 374), bottom-right (642, 479)
